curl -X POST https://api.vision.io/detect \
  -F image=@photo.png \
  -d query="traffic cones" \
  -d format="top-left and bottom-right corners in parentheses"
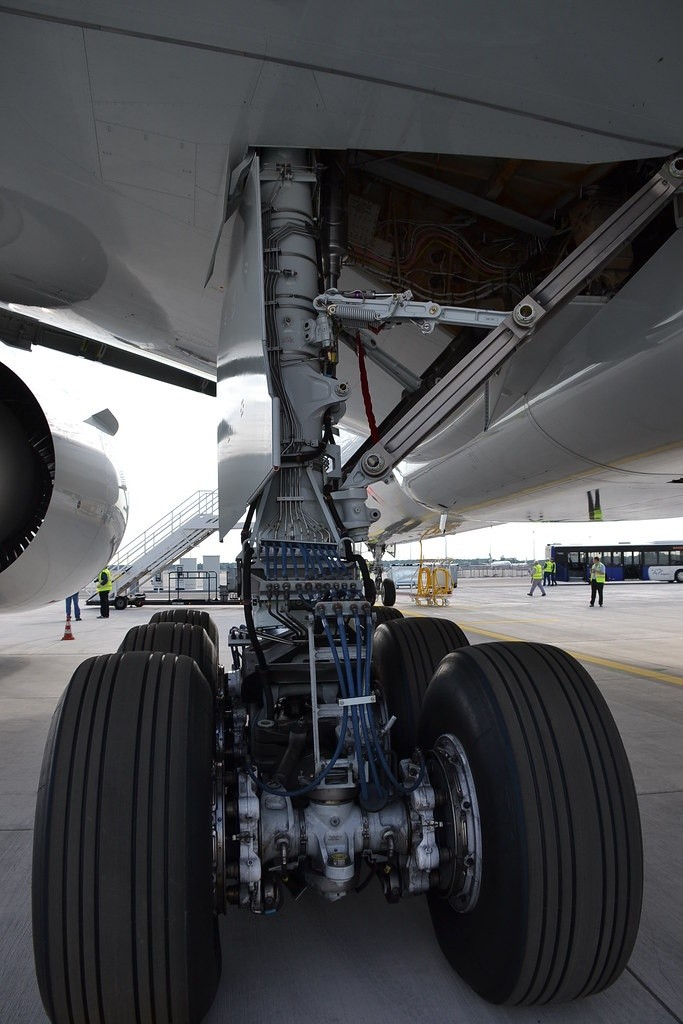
top-left (61, 615), bottom-right (74, 640)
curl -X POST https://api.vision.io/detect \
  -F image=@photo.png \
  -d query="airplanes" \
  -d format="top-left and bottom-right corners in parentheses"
top-left (0, 0), bottom-right (683, 1024)
top-left (470, 559), bottom-right (528, 570)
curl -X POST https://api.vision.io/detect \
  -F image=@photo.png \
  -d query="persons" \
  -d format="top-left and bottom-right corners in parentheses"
top-left (65, 592), bottom-right (82, 622)
top-left (587, 556), bottom-right (606, 608)
top-left (93, 565), bottom-right (113, 619)
top-left (543, 558), bottom-right (558, 586)
top-left (585, 488), bottom-right (603, 520)
top-left (526, 560), bottom-right (547, 597)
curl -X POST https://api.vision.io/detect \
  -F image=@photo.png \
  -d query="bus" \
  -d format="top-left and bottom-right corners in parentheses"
top-left (546, 541), bottom-right (683, 584)
